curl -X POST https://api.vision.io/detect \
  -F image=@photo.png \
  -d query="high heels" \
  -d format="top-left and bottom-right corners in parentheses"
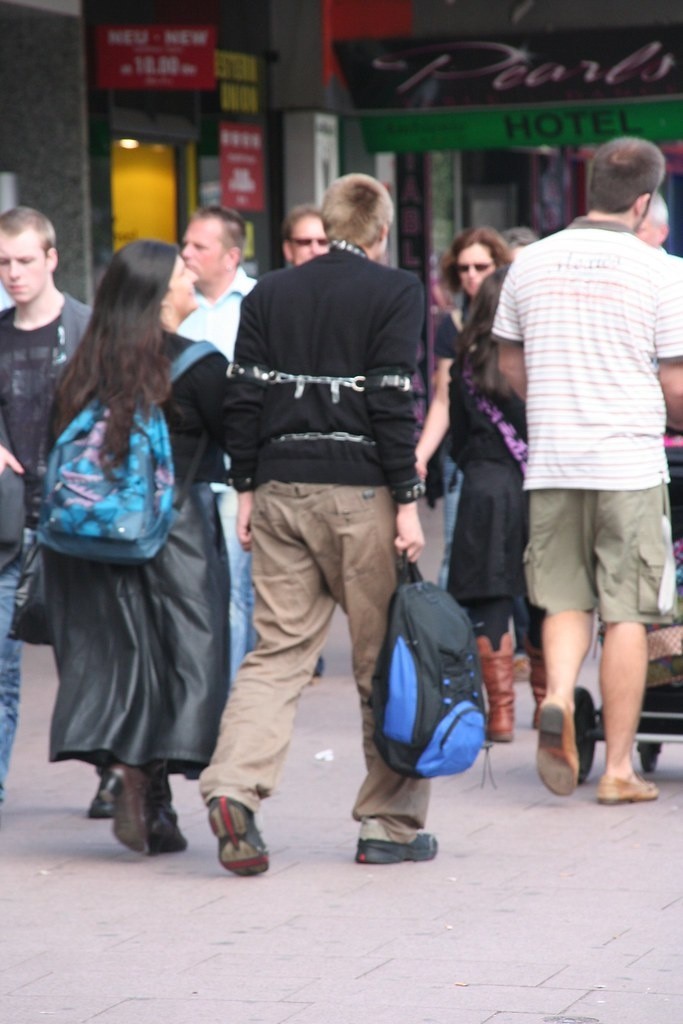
top-left (99, 759), bottom-right (190, 859)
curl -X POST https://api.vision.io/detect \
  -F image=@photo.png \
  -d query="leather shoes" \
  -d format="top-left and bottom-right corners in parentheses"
top-left (536, 697), bottom-right (578, 798)
top-left (89, 782), bottom-right (115, 817)
top-left (595, 770), bottom-right (659, 806)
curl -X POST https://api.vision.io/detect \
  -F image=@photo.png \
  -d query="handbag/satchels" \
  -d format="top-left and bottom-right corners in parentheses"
top-left (6, 539), bottom-right (52, 649)
top-left (596, 538), bottom-right (682, 686)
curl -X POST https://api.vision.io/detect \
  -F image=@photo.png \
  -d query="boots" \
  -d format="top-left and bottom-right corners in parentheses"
top-left (474, 631), bottom-right (514, 742)
top-left (522, 631), bottom-right (547, 729)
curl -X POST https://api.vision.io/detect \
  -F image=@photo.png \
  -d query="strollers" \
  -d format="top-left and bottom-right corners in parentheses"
top-left (569, 417), bottom-right (681, 786)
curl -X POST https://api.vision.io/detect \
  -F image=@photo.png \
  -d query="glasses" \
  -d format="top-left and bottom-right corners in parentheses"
top-left (286, 236), bottom-right (329, 247)
top-left (454, 259), bottom-right (494, 273)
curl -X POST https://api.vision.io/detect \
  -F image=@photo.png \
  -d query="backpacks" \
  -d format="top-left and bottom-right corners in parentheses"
top-left (368, 549), bottom-right (488, 779)
top-left (37, 339), bottom-right (223, 567)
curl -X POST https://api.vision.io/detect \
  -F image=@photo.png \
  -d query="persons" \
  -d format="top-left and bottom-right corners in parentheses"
top-left (416, 225), bottom-right (515, 598)
top-left (198, 172), bottom-right (488, 877)
top-left (450, 261), bottom-right (561, 745)
top-left (181, 211), bottom-right (257, 694)
top-left (633, 192), bottom-right (668, 249)
top-left (493, 136), bottom-right (681, 809)
top-left (1, 207), bottom-right (124, 823)
top-left (35, 246), bottom-right (236, 855)
top-left (278, 203), bottom-right (329, 268)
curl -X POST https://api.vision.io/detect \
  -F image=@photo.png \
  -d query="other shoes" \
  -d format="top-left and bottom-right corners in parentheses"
top-left (208, 796), bottom-right (269, 877)
top-left (354, 816), bottom-right (439, 865)
